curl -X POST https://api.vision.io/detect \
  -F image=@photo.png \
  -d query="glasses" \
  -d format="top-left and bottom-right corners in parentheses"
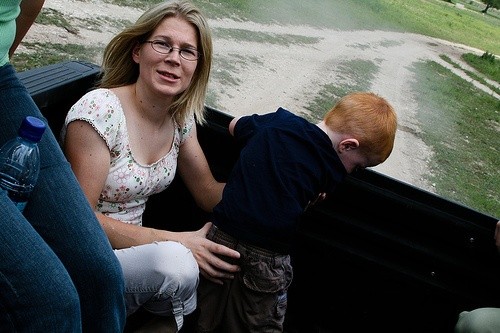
top-left (139, 39), bottom-right (203, 62)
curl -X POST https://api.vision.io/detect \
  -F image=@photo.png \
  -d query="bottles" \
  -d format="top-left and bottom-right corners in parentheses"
top-left (0, 115), bottom-right (48, 213)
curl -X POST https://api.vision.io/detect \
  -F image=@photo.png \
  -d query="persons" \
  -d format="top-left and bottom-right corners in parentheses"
top-left (1, 1), bottom-right (126, 333)
top-left (196, 93), bottom-right (397, 333)
top-left (59, 1), bottom-right (241, 333)
top-left (455, 220), bottom-right (500, 333)
